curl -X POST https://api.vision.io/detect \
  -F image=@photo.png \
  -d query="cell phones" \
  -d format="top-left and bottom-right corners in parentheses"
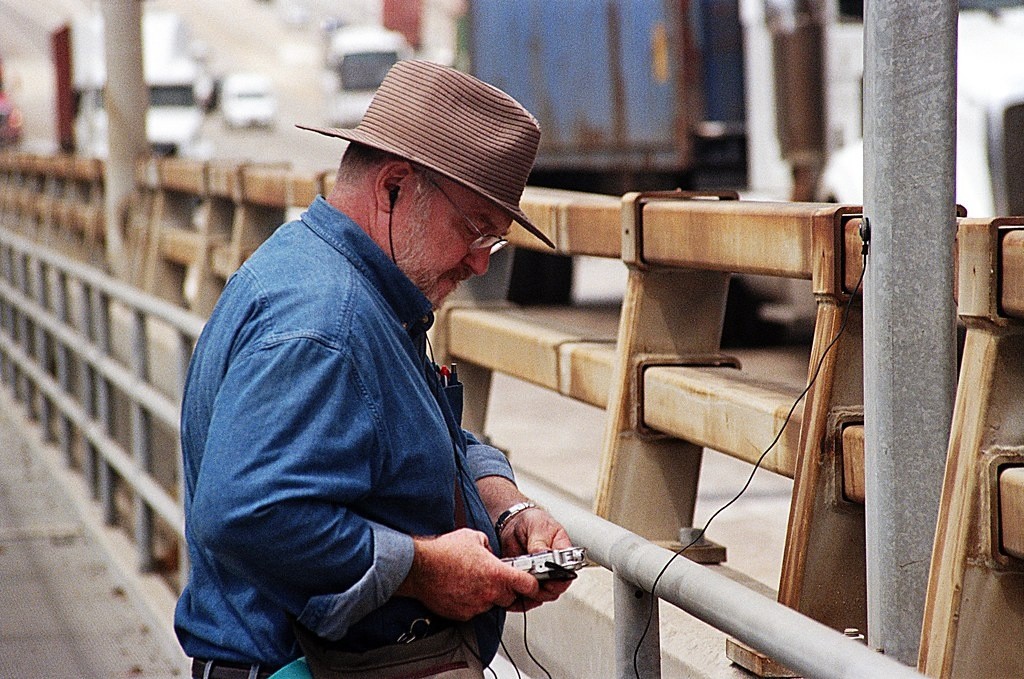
top-left (494, 549), bottom-right (590, 576)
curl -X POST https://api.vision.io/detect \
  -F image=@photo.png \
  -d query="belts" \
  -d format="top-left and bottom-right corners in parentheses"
top-left (190, 658), bottom-right (276, 679)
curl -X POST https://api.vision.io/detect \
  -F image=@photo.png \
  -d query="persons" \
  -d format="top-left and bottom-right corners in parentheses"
top-left (174, 61), bottom-right (576, 679)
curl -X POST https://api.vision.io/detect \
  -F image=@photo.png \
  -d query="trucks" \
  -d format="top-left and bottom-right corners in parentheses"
top-left (467, 1), bottom-right (754, 339)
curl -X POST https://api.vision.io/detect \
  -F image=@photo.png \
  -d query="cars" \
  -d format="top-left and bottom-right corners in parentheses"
top-left (75, 66), bottom-right (205, 160)
top-left (0, 61), bottom-right (23, 150)
top-left (222, 82), bottom-right (272, 128)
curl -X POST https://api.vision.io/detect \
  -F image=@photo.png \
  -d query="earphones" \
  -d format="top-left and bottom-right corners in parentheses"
top-left (389, 186), bottom-right (400, 207)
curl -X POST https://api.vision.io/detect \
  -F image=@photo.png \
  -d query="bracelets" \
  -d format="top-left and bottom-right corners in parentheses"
top-left (491, 501), bottom-right (546, 542)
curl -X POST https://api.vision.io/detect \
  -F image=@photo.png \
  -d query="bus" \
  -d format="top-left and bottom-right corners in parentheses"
top-left (326, 24), bottom-right (415, 128)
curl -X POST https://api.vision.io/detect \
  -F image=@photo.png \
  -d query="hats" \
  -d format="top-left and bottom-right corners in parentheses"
top-left (292, 56), bottom-right (559, 250)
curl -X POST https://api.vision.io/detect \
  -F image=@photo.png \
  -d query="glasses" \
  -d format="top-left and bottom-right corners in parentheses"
top-left (414, 168), bottom-right (509, 255)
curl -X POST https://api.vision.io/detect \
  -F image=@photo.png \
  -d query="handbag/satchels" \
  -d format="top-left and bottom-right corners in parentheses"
top-left (289, 613), bottom-right (485, 679)
